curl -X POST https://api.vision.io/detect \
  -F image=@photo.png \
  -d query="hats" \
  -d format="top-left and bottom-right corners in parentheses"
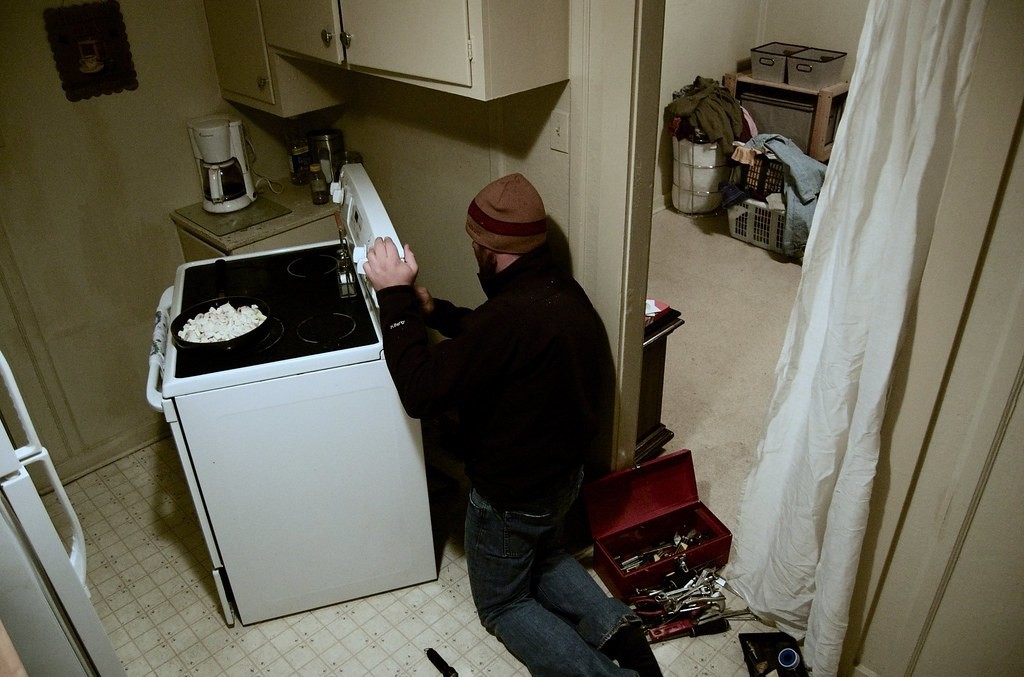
top-left (465, 172), bottom-right (547, 255)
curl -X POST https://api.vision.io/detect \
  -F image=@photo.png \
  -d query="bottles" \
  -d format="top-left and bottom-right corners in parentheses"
top-left (309, 163), bottom-right (329, 205)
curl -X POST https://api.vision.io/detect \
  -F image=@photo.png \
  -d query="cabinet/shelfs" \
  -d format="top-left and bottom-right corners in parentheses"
top-left (203, 0), bottom-right (570, 119)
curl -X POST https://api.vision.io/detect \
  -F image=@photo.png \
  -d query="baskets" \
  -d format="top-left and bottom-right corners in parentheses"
top-left (726, 150), bottom-right (786, 202)
top-left (726, 195), bottom-right (787, 253)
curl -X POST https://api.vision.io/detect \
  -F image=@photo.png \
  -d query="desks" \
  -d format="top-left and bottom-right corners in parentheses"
top-left (170, 179), bottom-right (352, 261)
top-left (728, 69), bottom-right (853, 163)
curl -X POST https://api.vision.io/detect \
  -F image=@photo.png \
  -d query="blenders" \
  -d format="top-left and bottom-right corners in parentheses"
top-left (187, 113), bottom-right (258, 213)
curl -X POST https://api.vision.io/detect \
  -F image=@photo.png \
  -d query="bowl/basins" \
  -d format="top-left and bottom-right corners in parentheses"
top-left (171, 297), bottom-right (272, 356)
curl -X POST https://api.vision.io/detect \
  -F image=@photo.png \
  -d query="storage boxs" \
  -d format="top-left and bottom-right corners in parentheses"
top-left (588, 446), bottom-right (733, 603)
top-left (724, 41), bottom-right (848, 255)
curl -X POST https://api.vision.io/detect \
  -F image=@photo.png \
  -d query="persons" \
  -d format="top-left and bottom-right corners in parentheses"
top-left (363, 172), bottom-right (663, 677)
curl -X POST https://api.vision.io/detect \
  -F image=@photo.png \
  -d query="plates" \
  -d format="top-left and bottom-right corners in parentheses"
top-left (171, 318), bottom-right (284, 359)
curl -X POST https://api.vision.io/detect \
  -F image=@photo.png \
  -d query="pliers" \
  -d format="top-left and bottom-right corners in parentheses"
top-left (619, 588), bottom-right (682, 617)
